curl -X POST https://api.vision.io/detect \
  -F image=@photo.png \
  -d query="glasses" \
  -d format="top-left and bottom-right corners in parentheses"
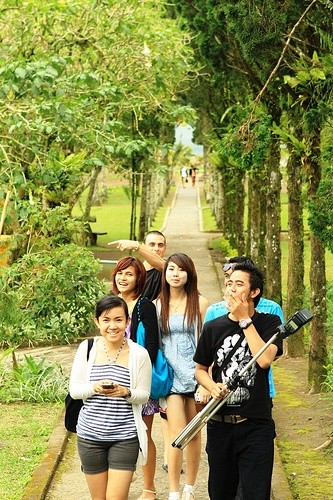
top-left (222, 264), bottom-right (237, 272)
top-left (149, 243), bottom-right (165, 248)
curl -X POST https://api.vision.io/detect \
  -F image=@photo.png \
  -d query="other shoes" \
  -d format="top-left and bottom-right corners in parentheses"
top-left (137, 489), bottom-right (156, 500)
top-left (161, 463), bottom-right (183, 474)
top-left (169, 493), bottom-right (195, 500)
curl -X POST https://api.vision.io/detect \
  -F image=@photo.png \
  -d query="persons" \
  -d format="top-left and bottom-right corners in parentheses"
top-left (69, 295), bottom-right (150, 500)
top-left (108, 230), bottom-right (168, 422)
top-left (148, 253), bottom-right (210, 499)
top-left (177, 164), bottom-right (199, 188)
top-left (106, 256), bottom-right (158, 499)
top-left (191, 264), bottom-right (284, 499)
top-left (203, 255), bottom-right (285, 500)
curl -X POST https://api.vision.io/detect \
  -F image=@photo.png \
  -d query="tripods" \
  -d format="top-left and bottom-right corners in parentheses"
top-left (171, 323), bottom-right (291, 451)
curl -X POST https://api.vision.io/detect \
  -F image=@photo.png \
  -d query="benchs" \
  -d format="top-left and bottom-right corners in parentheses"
top-left (87, 232), bottom-right (108, 245)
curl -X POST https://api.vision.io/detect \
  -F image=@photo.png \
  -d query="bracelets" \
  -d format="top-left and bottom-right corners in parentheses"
top-left (121, 388), bottom-right (131, 398)
top-left (133, 240), bottom-right (143, 252)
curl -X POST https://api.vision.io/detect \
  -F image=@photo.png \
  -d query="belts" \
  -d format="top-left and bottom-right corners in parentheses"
top-left (209, 413), bottom-right (249, 424)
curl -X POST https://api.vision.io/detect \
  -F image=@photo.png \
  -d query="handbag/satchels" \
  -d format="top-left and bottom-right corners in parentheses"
top-left (136, 297), bottom-right (174, 400)
top-left (64, 337), bottom-right (94, 433)
top-left (186, 179), bottom-right (188, 183)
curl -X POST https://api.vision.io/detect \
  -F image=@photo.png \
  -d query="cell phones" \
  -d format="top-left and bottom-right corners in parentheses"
top-left (102, 382), bottom-right (115, 389)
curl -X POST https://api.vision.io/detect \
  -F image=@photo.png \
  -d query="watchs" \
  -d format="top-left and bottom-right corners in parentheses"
top-left (238, 318), bottom-right (252, 328)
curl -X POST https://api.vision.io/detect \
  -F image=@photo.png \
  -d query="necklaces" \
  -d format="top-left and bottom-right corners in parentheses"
top-left (169, 292), bottom-right (187, 313)
top-left (102, 336), bottom-right (126, 365)
